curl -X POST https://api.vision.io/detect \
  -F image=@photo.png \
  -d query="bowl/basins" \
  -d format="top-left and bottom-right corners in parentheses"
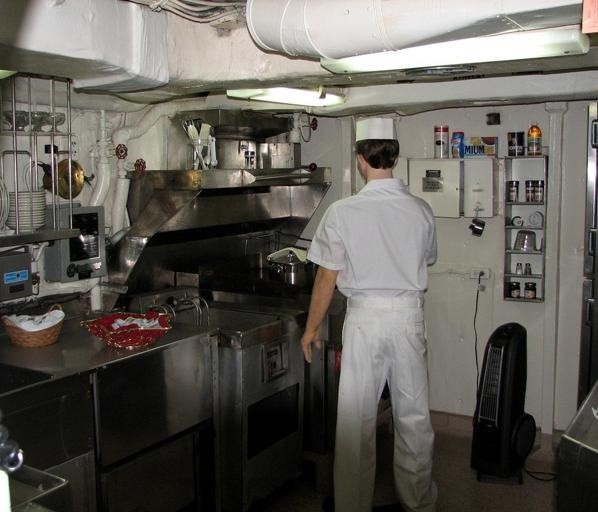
top-left (78, 311), bottom-right (173, 351)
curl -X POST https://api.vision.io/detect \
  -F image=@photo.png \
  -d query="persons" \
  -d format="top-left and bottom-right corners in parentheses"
top-left (297, 135), bottom-right (442, 512)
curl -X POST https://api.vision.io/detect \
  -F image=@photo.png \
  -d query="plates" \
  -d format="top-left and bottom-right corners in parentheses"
top-left (0, 177), bottom-right (47, 236)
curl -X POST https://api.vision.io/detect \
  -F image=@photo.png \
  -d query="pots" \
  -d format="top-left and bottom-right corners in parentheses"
top-left (268, 251), bottom-right (316, 287)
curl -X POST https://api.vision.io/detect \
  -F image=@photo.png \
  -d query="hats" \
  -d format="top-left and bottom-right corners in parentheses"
top-left (353, 117), bottom-right (397, 145)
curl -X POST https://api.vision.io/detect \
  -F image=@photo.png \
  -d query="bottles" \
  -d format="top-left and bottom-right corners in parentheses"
top-left (451, 132), bottom-right (464, 159)
top-left (433, 125), bottom-right (450, 158)
top-left (525, 180), bottom-right (544, 202)
top-left (508, 180), bottom-right (519, 201)
top-left (527, 121), bottom-right (542, 155)
top-left (508, 131), bottom-right (524, 156)
top-left (515, 262), bottom-right (531, 276)
top-left (186, 139), bottom-right (211, 171)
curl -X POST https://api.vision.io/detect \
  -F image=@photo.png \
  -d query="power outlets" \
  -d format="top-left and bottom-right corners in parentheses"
top-left (470, 268), bottom-right (491, 279)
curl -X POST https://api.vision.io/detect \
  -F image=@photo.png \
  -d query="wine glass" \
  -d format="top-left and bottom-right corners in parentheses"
top-left (4, 109), bottom-right (65, 133)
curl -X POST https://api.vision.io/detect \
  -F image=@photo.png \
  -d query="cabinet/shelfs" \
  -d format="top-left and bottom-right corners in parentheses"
top-left (0, 72), bottom-right (82, 246)
top-left (502, 155), bottom-right (548, 301)
top-left (0, 330), bottom-right (222, 510)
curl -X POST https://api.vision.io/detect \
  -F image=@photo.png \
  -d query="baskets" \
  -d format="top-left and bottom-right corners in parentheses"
top-left (1, 300), bottom-right (68, 351)
